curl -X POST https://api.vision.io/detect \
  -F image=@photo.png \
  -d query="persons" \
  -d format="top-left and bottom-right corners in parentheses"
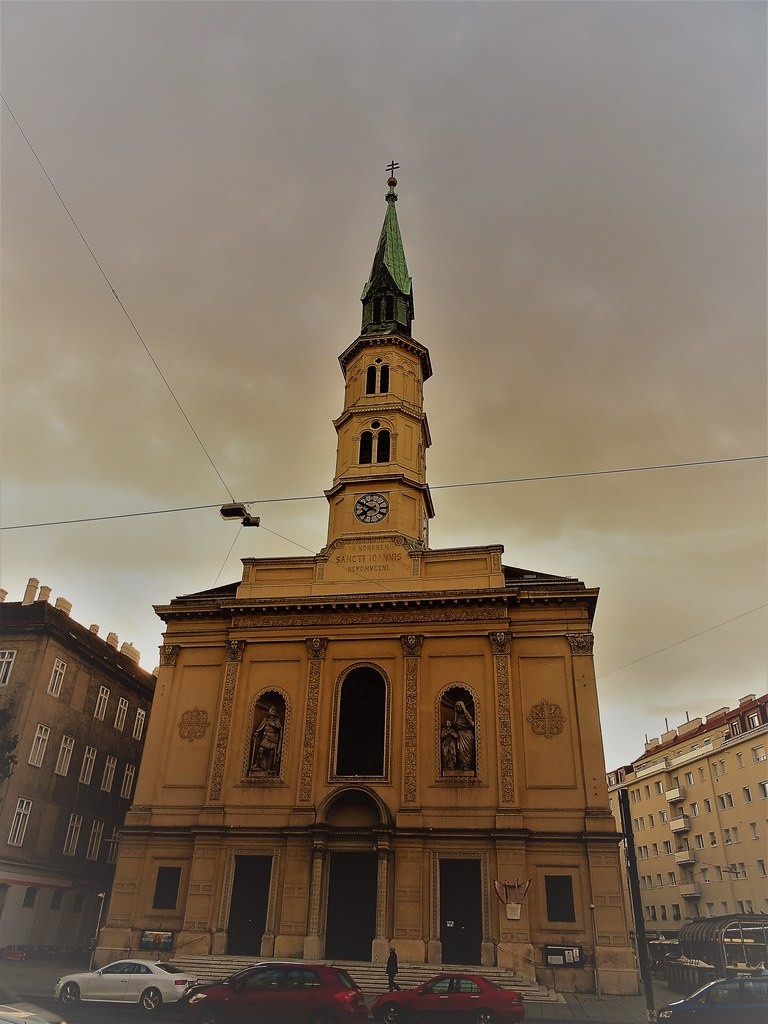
top-left (746, 963), bottom-right (751, 968)
top-left (732, 961), bottom-right (738, 967)
top-left (442, 698), bottom-right (475, 771)
top-left (385, 947), bottom-right (400, 992)
top-left (250, 704), bottom-right (281, 777)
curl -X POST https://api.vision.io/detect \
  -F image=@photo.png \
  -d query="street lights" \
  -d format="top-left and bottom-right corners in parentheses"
top-left (88, 891), bottom-right (106, 974)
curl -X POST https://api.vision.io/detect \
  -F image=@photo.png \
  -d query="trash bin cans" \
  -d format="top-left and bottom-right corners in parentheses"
top-left (667, 960), bottom-right (715, 996)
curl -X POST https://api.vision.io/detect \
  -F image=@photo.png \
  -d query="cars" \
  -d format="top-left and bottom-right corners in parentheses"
top-left (655, 971), bottom-right (768, 1024)
top-left (3, 941), bottom-right (91, 961)
top-left (52, 958), bottom-right (200, 1013)
top-left (370, 971), bottom-right (526, 1024)
top-left (182, 960), bottom-right (370, 1024)
